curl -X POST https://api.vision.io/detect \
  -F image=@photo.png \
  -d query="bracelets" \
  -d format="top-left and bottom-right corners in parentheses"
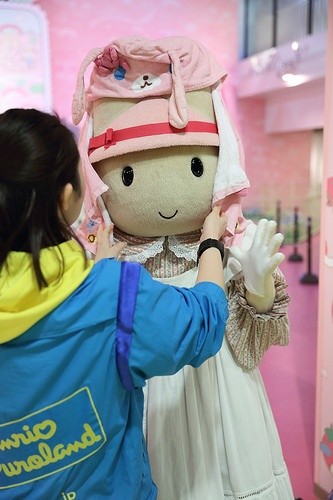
top-left (196, 237), bottom-right (225, 267)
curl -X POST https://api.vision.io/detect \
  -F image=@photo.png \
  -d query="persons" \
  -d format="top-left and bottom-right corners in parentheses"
top-left (0, 107), bottom-right (229, 500)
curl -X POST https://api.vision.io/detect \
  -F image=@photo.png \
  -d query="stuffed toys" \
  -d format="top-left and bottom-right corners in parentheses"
top-left (66, 34), bottom-right (298, 500)
